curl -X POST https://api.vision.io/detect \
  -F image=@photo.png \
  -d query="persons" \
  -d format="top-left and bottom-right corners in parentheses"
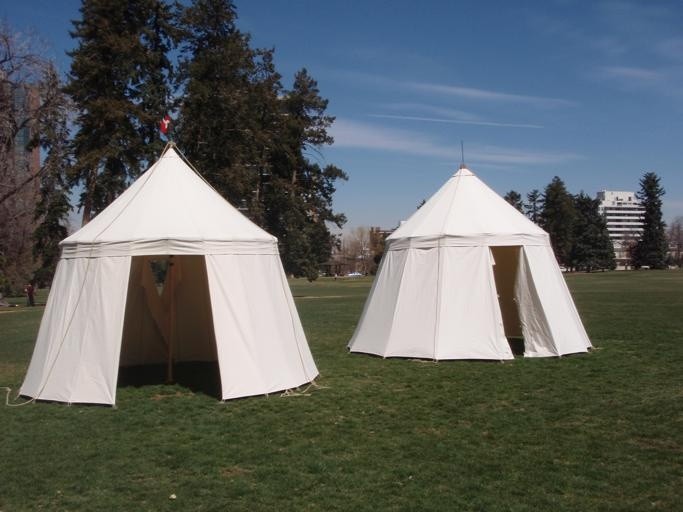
top-left (24, 283), bottom-right (36, 306)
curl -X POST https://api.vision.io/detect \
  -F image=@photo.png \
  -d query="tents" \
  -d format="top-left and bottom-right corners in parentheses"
top-left (346, 164), bottom-right (594, 362)
top-left (17, 141), bottom-right (319, 406)
